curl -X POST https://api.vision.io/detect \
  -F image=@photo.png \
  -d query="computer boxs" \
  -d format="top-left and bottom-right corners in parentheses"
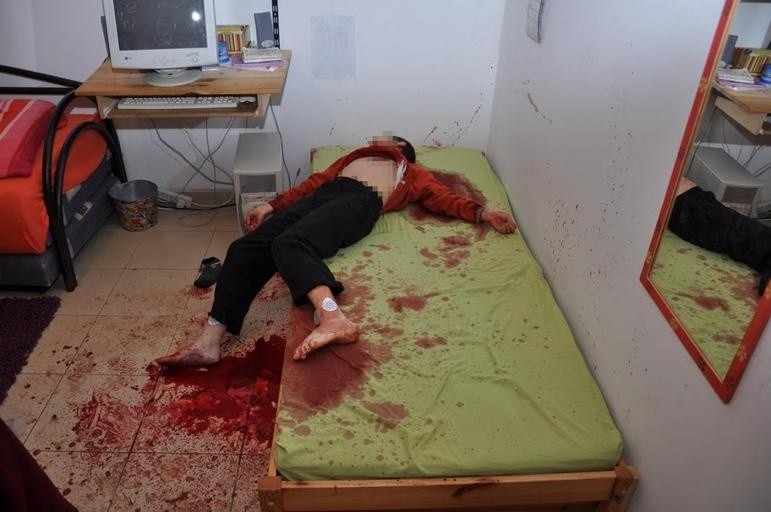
top-left (231, 133), bottom-right (285, 239)
top-left (686, 145), bottom-right (762, 220)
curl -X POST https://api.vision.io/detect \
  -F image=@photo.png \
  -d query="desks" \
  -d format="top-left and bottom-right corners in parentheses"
top-left (712, 79), bottom-right (771, 136)
top-left (74, 48), bottom-right (292, 120)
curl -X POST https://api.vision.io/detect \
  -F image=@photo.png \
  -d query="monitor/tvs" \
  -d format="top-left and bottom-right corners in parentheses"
top-left (102, 0), bottom-right (219, 70)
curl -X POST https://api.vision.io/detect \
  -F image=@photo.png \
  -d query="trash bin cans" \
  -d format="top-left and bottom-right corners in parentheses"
top-left (108, 179), bottom-right (159, 233)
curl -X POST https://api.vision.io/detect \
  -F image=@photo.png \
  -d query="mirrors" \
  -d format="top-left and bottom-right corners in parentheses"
top-left (639, 1), bottom-right (767, 404)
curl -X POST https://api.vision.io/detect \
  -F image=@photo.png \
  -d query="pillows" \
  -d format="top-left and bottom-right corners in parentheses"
top-left (0, 97), bottom-right (65, 178)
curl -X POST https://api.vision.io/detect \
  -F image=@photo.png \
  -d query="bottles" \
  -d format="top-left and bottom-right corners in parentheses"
top-left (760, 64), bottom-right (771, 86)
top-left (216, 41), bottom-right (229, 64)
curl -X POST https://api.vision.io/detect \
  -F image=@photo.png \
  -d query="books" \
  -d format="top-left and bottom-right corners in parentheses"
top-left (241, 46), bottom-right (282, 64)
top-left (733, 47), bottom-right (771, 76)
top-left (718, 68), bottom-right (755, 85)
top-left (216, 25), bottom-right (251, 54)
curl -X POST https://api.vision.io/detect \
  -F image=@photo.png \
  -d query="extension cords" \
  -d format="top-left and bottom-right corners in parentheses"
top-left (158, 188), bottom-right (193, 209)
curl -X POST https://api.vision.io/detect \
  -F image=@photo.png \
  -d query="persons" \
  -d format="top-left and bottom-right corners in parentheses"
top-left (155, 135), bottom-right (517, 368)
top-left (667, 175), bottom-right (770, 298)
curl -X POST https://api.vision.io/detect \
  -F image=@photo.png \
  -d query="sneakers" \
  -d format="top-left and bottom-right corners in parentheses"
top-left (194, 256), bottom-right (221, 287)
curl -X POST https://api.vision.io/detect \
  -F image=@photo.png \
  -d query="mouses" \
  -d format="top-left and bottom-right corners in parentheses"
top-left (239, 95), bottom-right (256, 103)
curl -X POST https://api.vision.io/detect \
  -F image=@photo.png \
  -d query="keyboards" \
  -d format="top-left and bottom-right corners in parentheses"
top-left (117, 96), bottom-right (239, 110)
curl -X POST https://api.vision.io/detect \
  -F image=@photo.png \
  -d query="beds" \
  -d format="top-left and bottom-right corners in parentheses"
top-left (649, 231), bottom-right (762, 382)
top-left (0, 63), bottom-right (131, 292)
top-left (257, 145), bottom-right (641, 512)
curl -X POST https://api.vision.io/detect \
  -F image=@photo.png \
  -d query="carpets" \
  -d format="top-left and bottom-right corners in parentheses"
top-left (0, 297), bottom-right (62, 402)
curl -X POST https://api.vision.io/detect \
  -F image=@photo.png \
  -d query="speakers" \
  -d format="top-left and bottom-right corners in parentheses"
top-left (253, 12), bottom-right (275, 48)
